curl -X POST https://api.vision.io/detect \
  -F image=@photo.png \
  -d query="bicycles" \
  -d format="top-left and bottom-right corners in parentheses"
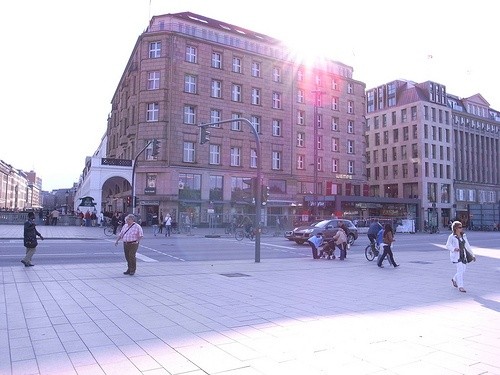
top-left (104, 223), bottom-right (124, 236)
top-left (364, 239), bottom-right (396, 261)
top-left (235, 225), bottom-right (257, 242)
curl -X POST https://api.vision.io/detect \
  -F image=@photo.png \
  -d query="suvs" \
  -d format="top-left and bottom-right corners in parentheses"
top-left (293, 219), bottom-right (358, 247)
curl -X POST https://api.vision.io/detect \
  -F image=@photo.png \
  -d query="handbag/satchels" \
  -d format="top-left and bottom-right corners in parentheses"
top-left (466, 251), bottom-right (473, 263)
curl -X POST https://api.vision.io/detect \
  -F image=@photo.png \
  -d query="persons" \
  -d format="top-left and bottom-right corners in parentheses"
top-left (333, 226), bottom-right (347, 261)
top-left (378, 223), bottom-right (399, 268)
top-left (21, 211), bottom-right (44, 267)
top-left (377, 224), bottom-right (393, 266)
top-left (307, 235), bottom-right (326, 259)
top-left (38, 206), bottom-right (128, 236)
top-left (238, 212), bottom-right (253, 239)
top-left (445, 221), bottom-right (476, 293)
top-left (152, 210), bottom-right (176, 238)
top-left (337, 221), bottom-right (349, 259)
top-left (114, 213), bottom-right (143, 275)
top-left (367, 221), bottom-right (384, 256)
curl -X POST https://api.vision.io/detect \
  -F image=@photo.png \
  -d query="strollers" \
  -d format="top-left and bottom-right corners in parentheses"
top-left (318, 239), bottom-right (336, 260)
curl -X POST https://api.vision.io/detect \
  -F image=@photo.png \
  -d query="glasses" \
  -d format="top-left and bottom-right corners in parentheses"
top-left (457, 227), bottom-right (463, 229)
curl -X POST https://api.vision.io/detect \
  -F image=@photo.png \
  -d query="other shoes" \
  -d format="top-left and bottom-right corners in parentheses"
top-left (309, 250), bottom-right (400, 268)
top-left (451, 278), bottom-right (466, 293)
top-left (123, 269), bottom-right (135, 276)
top-left (21, 259), bottom-right (34, 267)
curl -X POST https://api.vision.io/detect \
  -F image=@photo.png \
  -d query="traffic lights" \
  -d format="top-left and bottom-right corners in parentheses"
top-left (199, 122), bottom-right (210, 145)
top-left (152, 139), bottom-right (161, 156)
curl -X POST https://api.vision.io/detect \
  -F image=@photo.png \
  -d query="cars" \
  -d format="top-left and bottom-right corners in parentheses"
top-left (284, 219), bottom-right (323, 241)
